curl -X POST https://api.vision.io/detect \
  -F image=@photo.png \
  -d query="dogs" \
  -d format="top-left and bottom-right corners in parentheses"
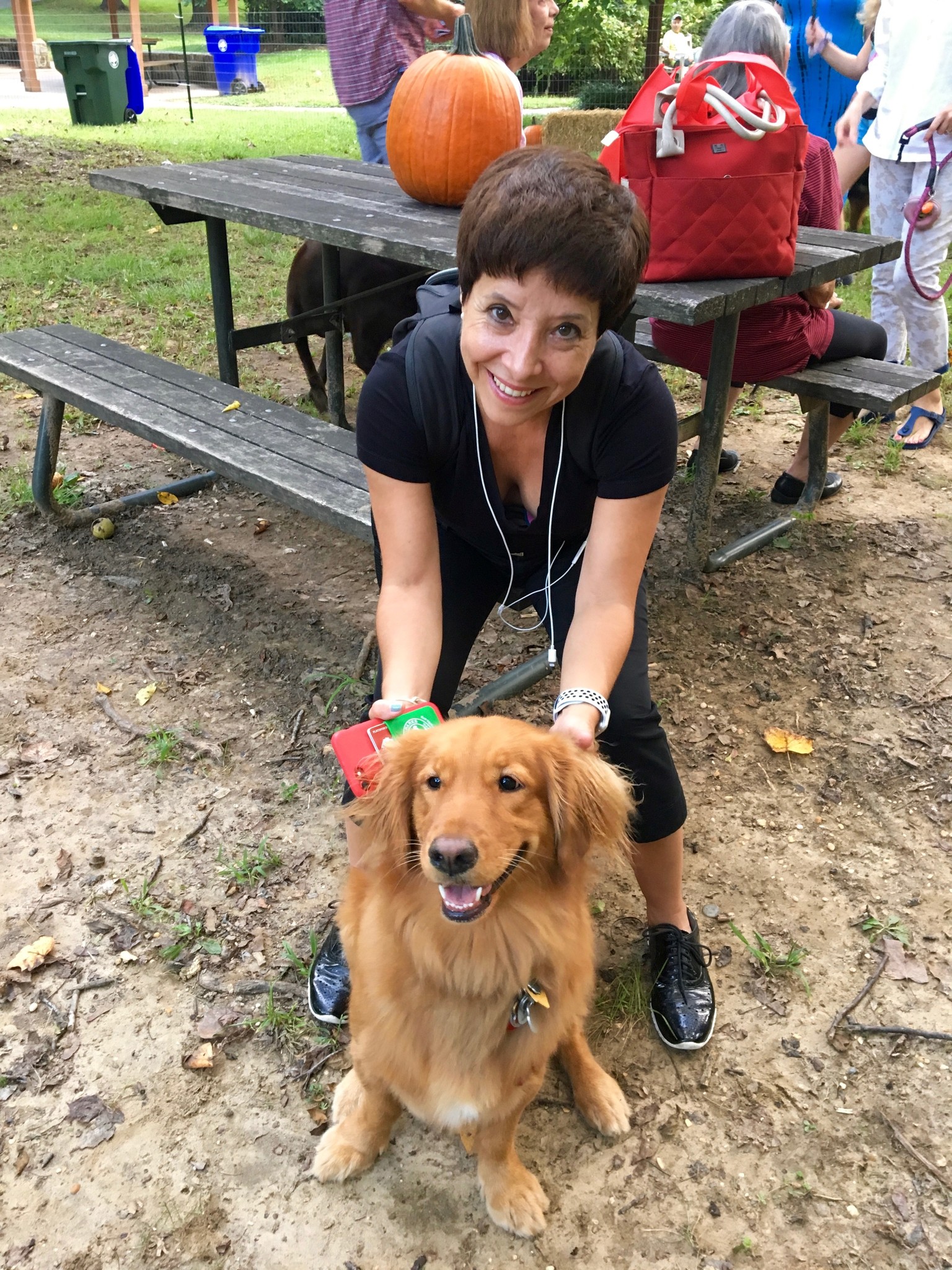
top-left (290, 240), bottom-right (435, 421)
top-left (310, 713), bottom-right (633, 1237)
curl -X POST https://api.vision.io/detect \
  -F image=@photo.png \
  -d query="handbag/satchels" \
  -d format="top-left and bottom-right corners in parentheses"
top-left (595, 51), bottom-right (809, 286)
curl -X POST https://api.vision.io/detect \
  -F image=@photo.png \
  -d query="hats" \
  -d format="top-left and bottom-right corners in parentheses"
top-left (671, 14), bottom-right (683, 20)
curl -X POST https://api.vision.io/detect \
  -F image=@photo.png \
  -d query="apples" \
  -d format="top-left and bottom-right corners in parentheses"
top-left (53, 472), bottom-right (63, 486)
top-left (91, 518), bottom-right (114, 538)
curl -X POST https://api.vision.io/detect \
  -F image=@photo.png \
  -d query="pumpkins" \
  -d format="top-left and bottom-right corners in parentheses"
top-left (523, 116), bottom-right (545, 146)
top-left (386, 13), bottom-right (522, 208)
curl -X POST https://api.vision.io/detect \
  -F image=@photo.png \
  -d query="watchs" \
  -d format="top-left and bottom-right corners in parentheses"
top-left (550, 687), bottom-right (611, 737)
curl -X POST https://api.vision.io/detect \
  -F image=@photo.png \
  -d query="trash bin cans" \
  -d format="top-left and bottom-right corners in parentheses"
top-left (205, 25), bottom-right (266, 95)
top-left (49, 41), bottom-right (144, 125)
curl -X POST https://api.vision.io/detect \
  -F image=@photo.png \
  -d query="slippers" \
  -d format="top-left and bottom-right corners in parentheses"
top-left (849, 404), bottom-right (896, 429)
top-left (887, 405), bottom-right (947, 449)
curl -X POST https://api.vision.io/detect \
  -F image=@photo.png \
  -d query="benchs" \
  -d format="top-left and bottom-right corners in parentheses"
top-left (0, 315), bottom-right (938, 718)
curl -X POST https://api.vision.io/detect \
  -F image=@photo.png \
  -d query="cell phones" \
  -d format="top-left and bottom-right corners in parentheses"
top-left (330, 701), bottom-right (447, 800)
top-left (812, 0), bottom-right (816, 24)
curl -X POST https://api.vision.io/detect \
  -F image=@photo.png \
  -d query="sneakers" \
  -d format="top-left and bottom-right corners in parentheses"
top-left (772, 470), bottom-right (842, 504)
top-left (641, 907), bottom-right (718, 1051)
top-left (305, 899), bottom-right (351, 1025)
top-left (686, 447), bottom-right (739, 473)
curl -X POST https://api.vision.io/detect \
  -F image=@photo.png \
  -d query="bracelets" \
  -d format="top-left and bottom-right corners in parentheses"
top-left (808, 30), bottom-right (832, 59)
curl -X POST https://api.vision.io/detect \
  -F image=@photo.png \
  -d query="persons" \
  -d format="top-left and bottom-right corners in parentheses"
top-left (661, 12), bottom-right (702, 68)
top-left (456, 0), bottom-right (563, 150)
top-left (834, 0), bottom-right (949, 455)
top-left (634, 0), bottom-right (890, 506)
top-left (316, 0), bottom-right (464, 169)
top-left (794, 0), bottom-right (887, 259)
top-left (308, 138), bottom-right (721, 1054)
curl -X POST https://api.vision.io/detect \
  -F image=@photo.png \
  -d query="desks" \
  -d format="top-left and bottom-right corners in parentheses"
top-left (89, 154), bottom-right (903, 718)
